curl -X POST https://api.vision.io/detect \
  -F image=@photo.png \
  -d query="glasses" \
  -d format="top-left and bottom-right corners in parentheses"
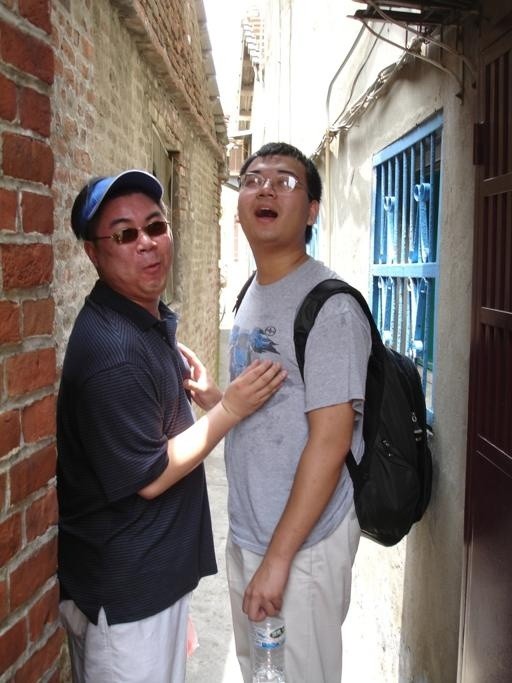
top-left (83, 219), bottom-right (170, 246)
top-left (235, 172), bottom-right (316, 199)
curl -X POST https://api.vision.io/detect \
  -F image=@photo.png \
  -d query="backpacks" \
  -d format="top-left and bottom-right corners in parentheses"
top-left (234, 271), bottom-right (432, 547)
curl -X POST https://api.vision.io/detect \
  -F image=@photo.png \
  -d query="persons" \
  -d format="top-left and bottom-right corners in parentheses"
top-left (174, 143), bottom-right (372, 682)
top-left (56, 169), bottom-right (290, 682)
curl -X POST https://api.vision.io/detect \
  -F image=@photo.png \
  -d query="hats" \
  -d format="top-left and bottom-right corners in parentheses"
top-left (70, 170), bottom-right (162, 237)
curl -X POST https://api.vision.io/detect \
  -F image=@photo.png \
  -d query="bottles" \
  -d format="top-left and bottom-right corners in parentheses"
top-left (249, 607), bottom-right (288, 682)
